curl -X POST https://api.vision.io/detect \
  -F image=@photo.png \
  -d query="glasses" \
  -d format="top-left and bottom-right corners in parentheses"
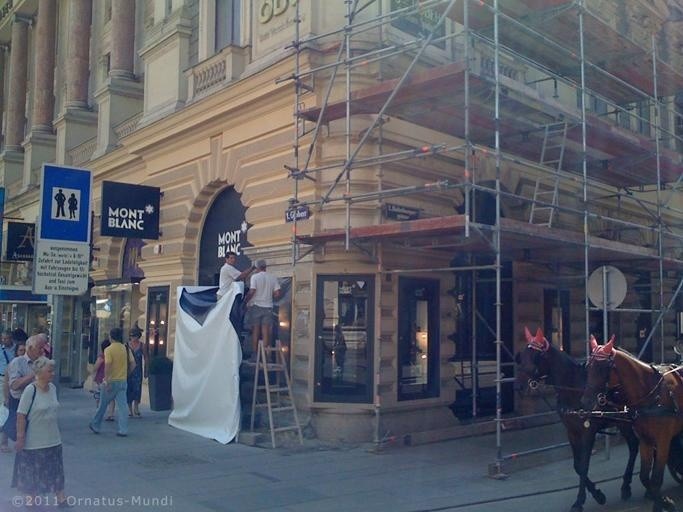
top-left (128, 333), bottom-right (139, 337)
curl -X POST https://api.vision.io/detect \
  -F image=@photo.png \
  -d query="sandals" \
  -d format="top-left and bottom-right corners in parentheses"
top-left (104, 415), bottom-right (115, 422)
top-left (0, 444), bottom-right (12, 452)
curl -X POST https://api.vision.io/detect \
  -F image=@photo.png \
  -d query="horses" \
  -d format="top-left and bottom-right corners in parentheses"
top-left (513, 326), bottom-right (657, 512)
top-left (577, 333), bottom-right (683, 512)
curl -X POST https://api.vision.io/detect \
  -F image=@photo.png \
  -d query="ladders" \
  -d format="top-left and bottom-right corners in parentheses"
top-left (527, 119), bottom-right (568, 229)
top-left (250, 340), bottom-right (304, 449)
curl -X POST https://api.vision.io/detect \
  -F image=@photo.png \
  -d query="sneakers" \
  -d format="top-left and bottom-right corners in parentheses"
top-left (247, 355), bottom-right (257, 362)
top-left (114, 430), bottom-right (128, 438)
top-left (56, 497), bottom-right (74, 508)
top-left (88, 421), bottom-right (100, 434)
top-left (265, 357), bottom-right (275, 363)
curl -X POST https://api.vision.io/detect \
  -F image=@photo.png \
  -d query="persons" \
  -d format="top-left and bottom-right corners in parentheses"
top-left (94, 339), bottom-right (116, 421)
top-left (219, 252), bottom-right (255, 315)
top-left (10, 356), bottom-right (72, 509)
top-left (124, 328), bottom-right (149, 418)
top-left (243, 259), bottom-right (281, 363)
top-left (0, 328), bottom-right (53, 453)
top-left (89, 328), bottom-right (136, 436)
top-left (331, 325), bottom-right (347, 372)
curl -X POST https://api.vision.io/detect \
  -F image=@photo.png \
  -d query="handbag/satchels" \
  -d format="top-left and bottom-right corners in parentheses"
top-left (2, 409), bottom-right (28, 442)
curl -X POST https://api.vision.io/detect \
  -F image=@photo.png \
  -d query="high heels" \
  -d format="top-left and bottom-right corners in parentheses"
top-left (133, 409), bottom-right (142, 417)
top-left (128, 410), bottom-right (133, 417)
